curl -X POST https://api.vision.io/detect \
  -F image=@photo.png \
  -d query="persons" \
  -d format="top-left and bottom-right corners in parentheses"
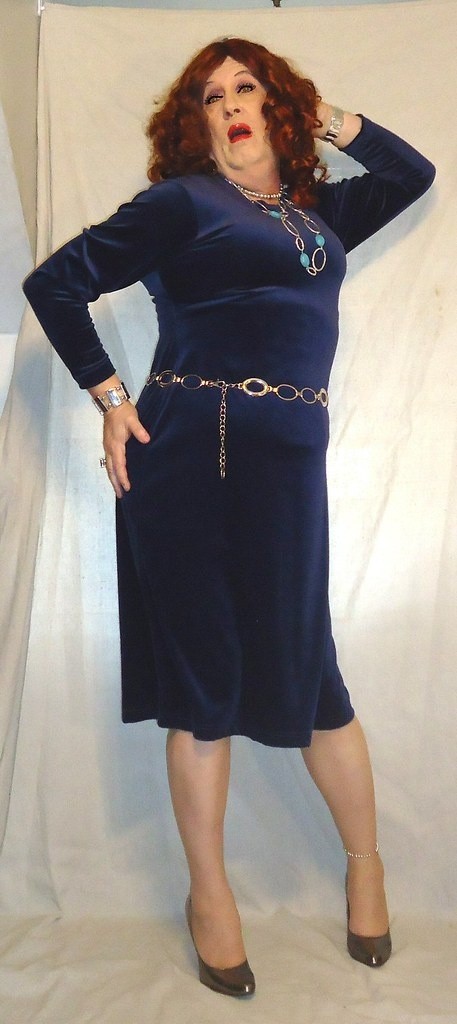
top-left (17, 36), bottom-right (435, 1002)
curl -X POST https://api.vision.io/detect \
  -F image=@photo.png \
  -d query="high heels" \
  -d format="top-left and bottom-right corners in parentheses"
top-left (185, 895), bottom-right (255, 996)
top-left (344, 873), bottom-right (392, 967)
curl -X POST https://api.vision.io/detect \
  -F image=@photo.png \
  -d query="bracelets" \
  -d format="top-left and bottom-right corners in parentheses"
top-left (318, 105), bottom-right (345, 142)
top-left (92, 379), bottom-right (131, 417)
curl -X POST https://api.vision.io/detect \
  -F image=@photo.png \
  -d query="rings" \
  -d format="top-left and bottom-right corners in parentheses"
top-left (99, 457), bottom-right (108, 469)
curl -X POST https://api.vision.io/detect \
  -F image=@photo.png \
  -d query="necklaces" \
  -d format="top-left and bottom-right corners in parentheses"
top-left (226, 177), bottom-right (327, 276)
